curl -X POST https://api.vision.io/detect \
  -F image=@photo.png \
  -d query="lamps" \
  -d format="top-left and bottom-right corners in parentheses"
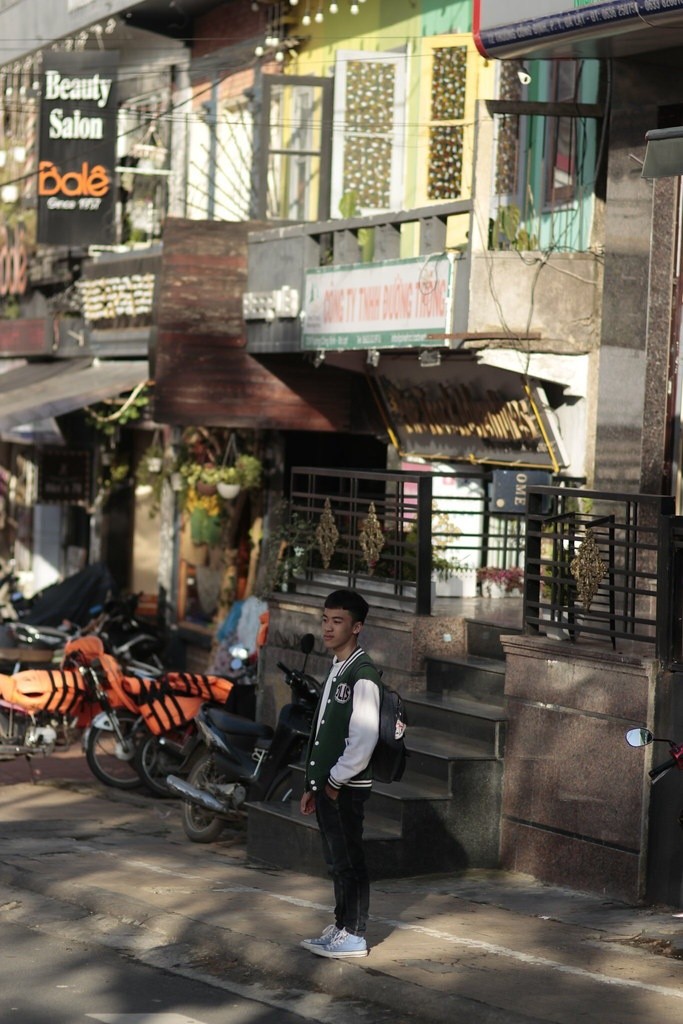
top-left (368, 349), bottom-right (380, 367)
top-left (516, 61), bottom-right (532, 84)
top-left (313, 351), bottom-right (326, 368)
top-left (417, 348), bottom-right (441, 367)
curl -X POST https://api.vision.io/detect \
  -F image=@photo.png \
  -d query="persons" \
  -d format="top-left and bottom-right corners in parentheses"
top-left (299, 589), bottom-right (383, 959)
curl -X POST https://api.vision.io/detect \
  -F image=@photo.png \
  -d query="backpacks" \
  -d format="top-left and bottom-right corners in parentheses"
top-left (346, 660), bottom-right (412, 784)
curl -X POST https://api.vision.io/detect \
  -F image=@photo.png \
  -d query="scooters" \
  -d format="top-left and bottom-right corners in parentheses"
top-left (0, 555), bottom-right (261, 802)
top-left (164, 632), bottom-right (387, 845)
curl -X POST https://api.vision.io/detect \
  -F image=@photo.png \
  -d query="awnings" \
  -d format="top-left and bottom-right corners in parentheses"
top-left (0, 359), bottom-right (149, 432)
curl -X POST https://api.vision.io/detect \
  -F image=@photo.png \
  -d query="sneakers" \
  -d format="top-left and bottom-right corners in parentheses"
top-left (308, 927), bottom-right (368, 960)
top-left (298, 922), bottom-right (342, 951)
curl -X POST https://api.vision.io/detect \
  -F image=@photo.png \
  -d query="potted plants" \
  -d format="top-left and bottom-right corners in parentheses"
top-left (179, 455), bottom-right (263, 499)
top-left (135, 446), bottom-right (172, 520)
